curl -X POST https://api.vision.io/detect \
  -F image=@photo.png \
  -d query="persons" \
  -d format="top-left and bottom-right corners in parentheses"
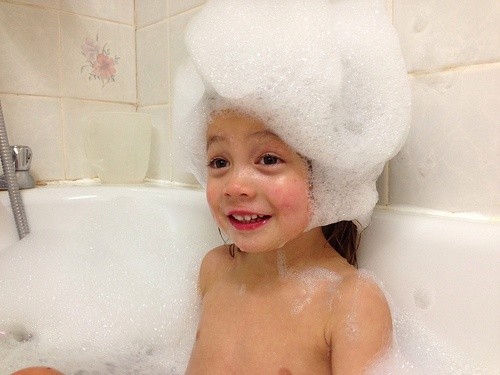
top-left (10, 107), bottom-right (392, 374)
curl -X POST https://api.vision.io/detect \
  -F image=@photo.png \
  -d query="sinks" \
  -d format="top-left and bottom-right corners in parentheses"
top-left (0, 183), bottom-right (500, 375)
